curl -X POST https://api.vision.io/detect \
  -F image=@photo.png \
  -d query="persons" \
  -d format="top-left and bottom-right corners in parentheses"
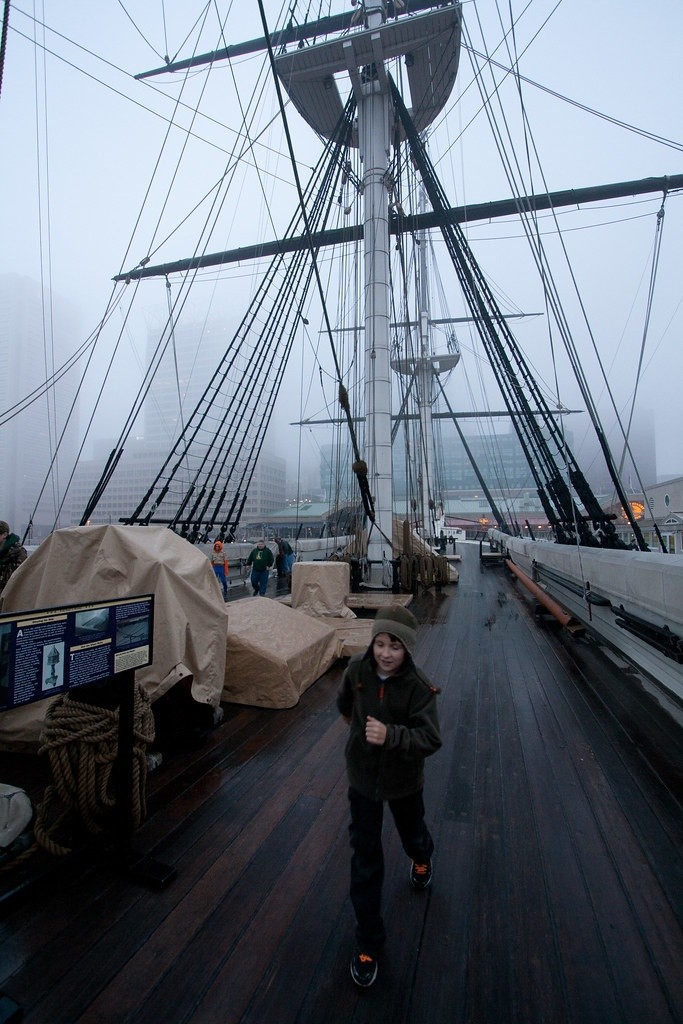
top-left (245, 540), bottom-right (274, 596)
top-left (211, 541), bottom-right (228, 596)
top-left (275, 538), bottom-right (294, 578)
top-left (628, 539), bottom-right (639, 551)
top-left (336, 604), bottom-right (443, 988)
top-left (0, 521), bottom-right (27, 594)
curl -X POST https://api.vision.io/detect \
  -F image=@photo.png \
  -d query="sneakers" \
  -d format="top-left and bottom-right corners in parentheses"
top-left (410, 857), bottom-right (432, 888)
top-left (351, 944), bottom-right (376, 986)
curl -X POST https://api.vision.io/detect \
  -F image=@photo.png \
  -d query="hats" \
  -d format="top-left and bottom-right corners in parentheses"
top-left (355, 603), bottom-right (442, 695)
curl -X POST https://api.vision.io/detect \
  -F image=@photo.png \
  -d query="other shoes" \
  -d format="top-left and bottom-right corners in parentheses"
top-left (253, 590), bottom-right (258, 596)
